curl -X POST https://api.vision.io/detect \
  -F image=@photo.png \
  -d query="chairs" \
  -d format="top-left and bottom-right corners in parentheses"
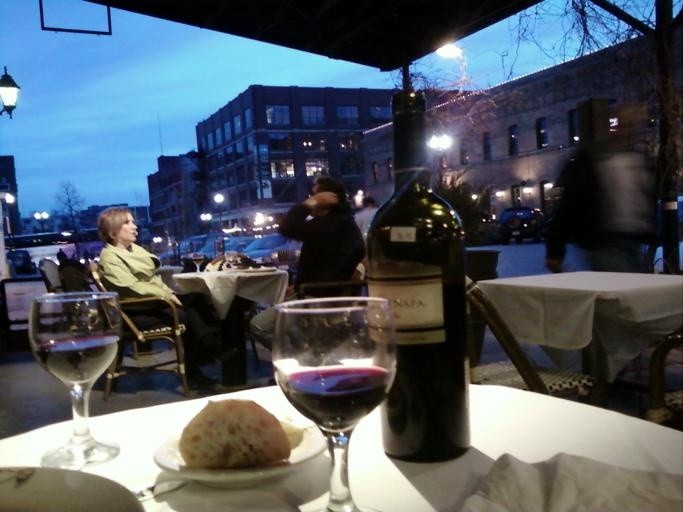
top-left (67, 247), bottom-right (189, 399)
top-left (36, 256), bottom-right (78, 325)
top-left (460, 272), bottom-right (594, 404)
top-left (637, 322), bottom-right (682, 428)
top-left (250, 260), bottom-right (374, 388)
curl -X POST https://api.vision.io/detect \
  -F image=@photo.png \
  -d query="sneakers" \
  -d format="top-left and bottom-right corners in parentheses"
top-left (207, 381), bottom-right (235, 393)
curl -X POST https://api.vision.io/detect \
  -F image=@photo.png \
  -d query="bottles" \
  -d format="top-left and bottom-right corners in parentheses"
top-left (362, 87), bottom-right (468, 461)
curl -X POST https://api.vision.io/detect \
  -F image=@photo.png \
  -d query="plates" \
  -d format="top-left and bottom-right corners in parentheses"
top-left (1, 465), bottom-right (149, 511)
top-left (150, 430), bottom-right (327, 489)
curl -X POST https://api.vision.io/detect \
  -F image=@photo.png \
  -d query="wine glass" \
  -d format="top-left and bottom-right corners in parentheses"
top-left (273, 296), bottom-right (400, 512)
top-left (188, 248), bottom-right (282, 274)
top-left (27, 291), bottom-right (123, 465)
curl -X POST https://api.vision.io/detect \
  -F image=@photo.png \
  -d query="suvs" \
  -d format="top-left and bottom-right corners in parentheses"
top-left (655, 197), bottom-right (682, 244)
top-left (498, 205), bottom-right (546, 247)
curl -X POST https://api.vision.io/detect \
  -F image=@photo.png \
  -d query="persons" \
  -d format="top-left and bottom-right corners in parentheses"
top-left (57, 248), bottom-right (95, 263)
top-left (544, 99), bottom-right (657, 272)
top-left (96, 205), bottom-right (208, 396)
top-left (249, 176), bottom-right (366, 385)
top-left (353, 196), bottom-right (379, 244)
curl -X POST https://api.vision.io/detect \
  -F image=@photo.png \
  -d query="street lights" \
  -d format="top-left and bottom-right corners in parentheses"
top-left (430, 133), bottom-right (452, 188)
top-left (0, 226), bottom-right (104, 268)
top-left (199, 212), bottom-right (212, 233)
top-left (33, 210), bottom-right (50, 234)
top-left (215, 194), bottom-right (225, 256)
top-left (0, 192), bottom-right (15, 220)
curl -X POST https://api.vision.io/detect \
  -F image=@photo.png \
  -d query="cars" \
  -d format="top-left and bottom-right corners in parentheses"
top-left (474, 215), bottom-right (496, 243)
top-left (156, 234), bottom-right (302, 265)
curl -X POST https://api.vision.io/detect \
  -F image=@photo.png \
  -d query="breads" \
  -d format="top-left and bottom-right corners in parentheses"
top-left (178, 398), bottom-right (292, 470)
